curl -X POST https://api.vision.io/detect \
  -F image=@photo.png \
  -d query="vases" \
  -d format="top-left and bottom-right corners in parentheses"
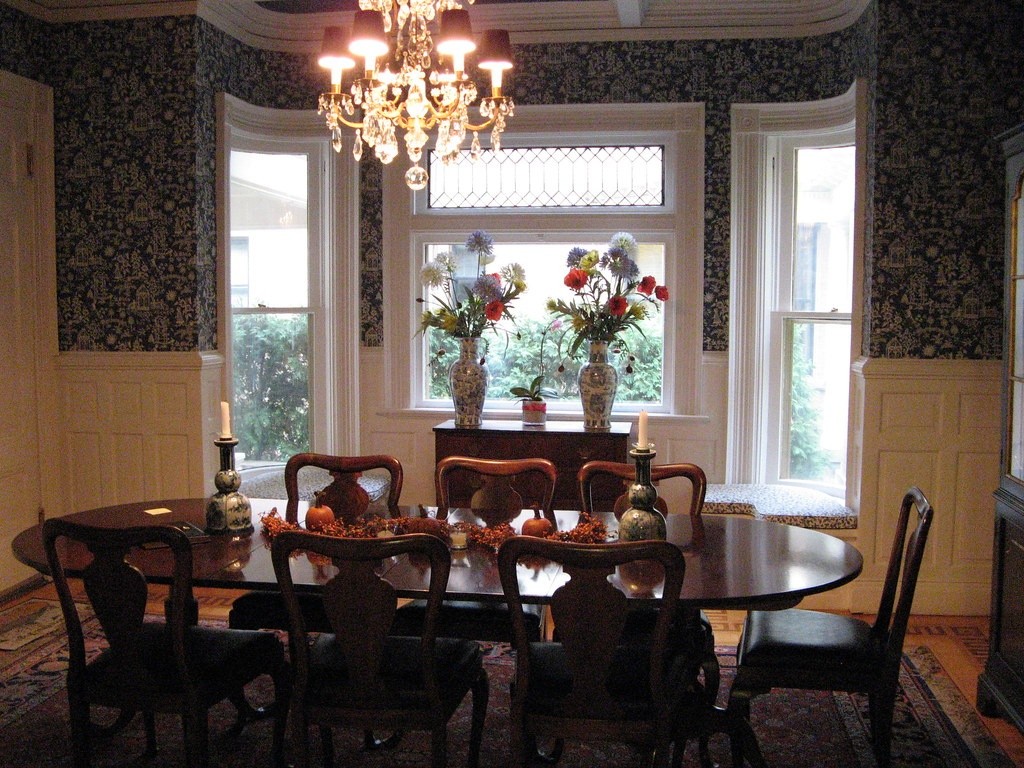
top-left (448, 341), bottom-right (484, 426)
top-left (577, 342), bottom-right (619, 430)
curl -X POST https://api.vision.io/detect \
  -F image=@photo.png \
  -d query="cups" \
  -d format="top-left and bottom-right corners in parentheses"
top-left (449, 530), bottom-right (468, 549)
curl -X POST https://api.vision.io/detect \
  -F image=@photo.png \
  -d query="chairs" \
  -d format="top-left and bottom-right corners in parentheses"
top-left (282, 454), bottom-right (404, 504)
top-left (436, 457), bottom-right (557, 511)
top-left (575, 457), bottom-right (708, 516)
top-left (41, 517), bottom-right (289, 768)
top-left (499, 534), bottom-right (713, 768)
top-left (266, 530), bottom-right (491, 768)
top-left (726, 486), bottom-right (934, 768)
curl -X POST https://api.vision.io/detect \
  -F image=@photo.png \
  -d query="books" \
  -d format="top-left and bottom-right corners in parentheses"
top-left (141, 520), bottom-right (210, 550)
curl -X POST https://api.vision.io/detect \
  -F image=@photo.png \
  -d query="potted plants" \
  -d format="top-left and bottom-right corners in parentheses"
top-left (508, 378), bottom-right (557, 425)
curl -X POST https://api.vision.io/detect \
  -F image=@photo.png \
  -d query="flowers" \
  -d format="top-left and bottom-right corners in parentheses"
top-left (546, 229), bottom-right (671, 355)
top-left (409, 228), bottom-right (527, 339)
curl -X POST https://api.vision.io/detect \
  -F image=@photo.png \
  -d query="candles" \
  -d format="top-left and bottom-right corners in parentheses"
top-left (220, 400), bottom-right (232, 435)
top-left (639, 409), bottom-right (650, 447)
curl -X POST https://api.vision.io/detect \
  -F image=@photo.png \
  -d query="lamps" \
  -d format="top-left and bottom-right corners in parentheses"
top-left (313, 0), bottom-right (517, 188)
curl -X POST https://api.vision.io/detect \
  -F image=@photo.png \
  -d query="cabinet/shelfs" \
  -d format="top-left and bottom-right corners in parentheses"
top-left (433, 418), bottom-right (632, 507)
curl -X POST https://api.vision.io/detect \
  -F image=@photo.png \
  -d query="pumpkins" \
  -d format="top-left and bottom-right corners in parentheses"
top-left (522, 504), bottom-right (553, 537)
top-left (305, 492), bottom-right (333, 528)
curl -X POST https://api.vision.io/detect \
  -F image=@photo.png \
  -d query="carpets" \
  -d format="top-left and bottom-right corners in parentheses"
top-left (0, 598), bottom-right (1018, 768)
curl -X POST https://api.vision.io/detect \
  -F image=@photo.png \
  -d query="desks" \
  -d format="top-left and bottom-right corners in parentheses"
top-left (12, 497), bottom-right (863, 768)
